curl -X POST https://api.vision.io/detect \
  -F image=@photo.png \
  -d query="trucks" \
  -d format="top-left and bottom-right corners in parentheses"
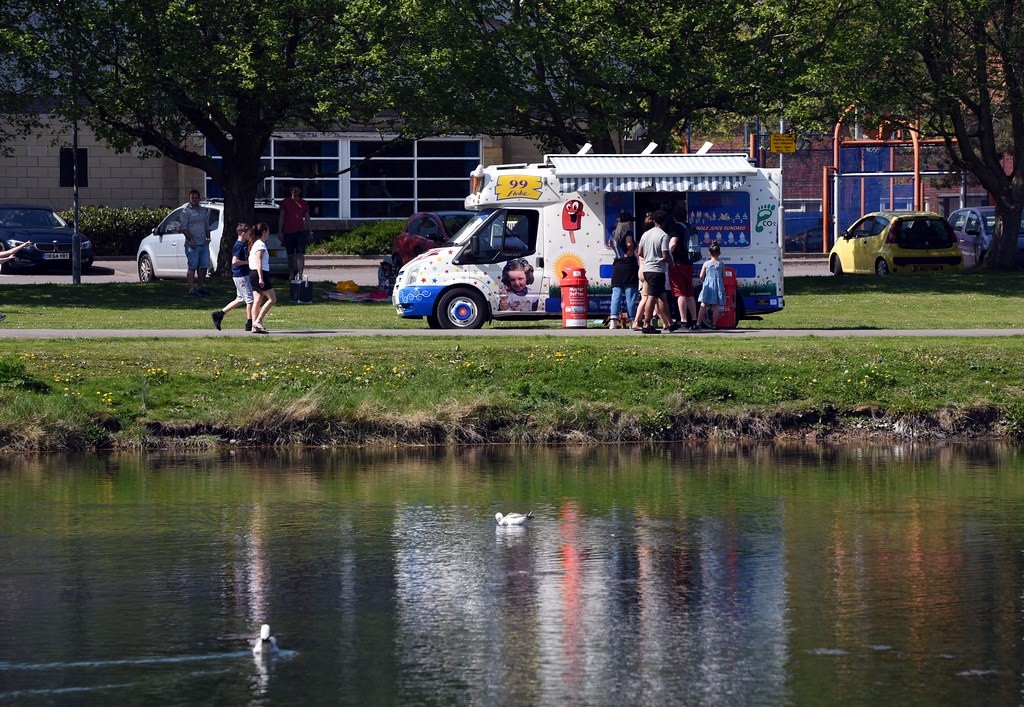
top-left (392, 142), bottom-right (785, 330)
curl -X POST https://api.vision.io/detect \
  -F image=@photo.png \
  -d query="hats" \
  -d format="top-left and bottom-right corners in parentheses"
top-left (617, 212), bottom-right (637, 223)
top-left (653, 210), bottom-right (666, 224)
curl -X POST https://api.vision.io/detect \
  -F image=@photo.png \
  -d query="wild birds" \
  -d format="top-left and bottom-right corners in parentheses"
top-left (495, 510), bottom-right (535, 526)
top-left (253, 624), bottom-right (281, 653)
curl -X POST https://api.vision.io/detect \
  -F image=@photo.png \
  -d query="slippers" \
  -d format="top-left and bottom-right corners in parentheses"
top-left (634, 324), bottom-right (655, 331)
top-left (663, 327), bottom-right (668, 330)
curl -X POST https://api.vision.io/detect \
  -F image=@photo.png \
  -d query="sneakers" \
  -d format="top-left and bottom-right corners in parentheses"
top-left (609, 322), bottom-right (621, 329)
top-left (252, 322), bottom-right (269, 334)
top-left (642, 326), bottom-right (661, 333)
top-left (212, 311), bottom-right (223, 331)
top-left (245, 324), bottom-right (252, 331)
top-left (668, 323), bottom-right (681, 333)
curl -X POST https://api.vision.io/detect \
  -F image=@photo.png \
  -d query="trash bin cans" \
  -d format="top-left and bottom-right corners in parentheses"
top-left (559, 267), bottom-right (588, 328)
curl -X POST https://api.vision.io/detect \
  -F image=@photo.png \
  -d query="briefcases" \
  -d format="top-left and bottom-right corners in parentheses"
top-left (290, 276), bottom-right (313, 303)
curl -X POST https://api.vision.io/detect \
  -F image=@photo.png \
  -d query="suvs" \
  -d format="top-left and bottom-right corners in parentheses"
top-left (137, 197), bottom-right (291, 283)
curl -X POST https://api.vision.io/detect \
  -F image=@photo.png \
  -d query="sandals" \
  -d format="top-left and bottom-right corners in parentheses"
top-left (689, 323), bottom-right (702, 330)
top-left (712, 324), bottom-right (721, 330)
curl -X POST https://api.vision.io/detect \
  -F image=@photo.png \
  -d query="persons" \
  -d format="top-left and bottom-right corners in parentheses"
top-left (498, 260), bottom-right (539, 311)
top-left (0, 240), bottom-right (32, 321)
top-left (181, 189), bottom-right (211, 297)
top-left (277, 186), bottom-right (313, 282)
top-left (378, 253), bottom-right (404, 296)
top-left (248, 223), bottom-right (277, 334)
top-left (632, 204), bottom-right (698, 334)
top-left (689, 240), bottom-right (726, 330)
top-left (609, 212), bottom-right (644, 331)
top-left (211, 223), bottom-right (254, 331)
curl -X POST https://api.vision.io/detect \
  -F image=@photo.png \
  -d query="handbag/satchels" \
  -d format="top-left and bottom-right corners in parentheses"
top-left (369, 291), bottom-right (388, 299)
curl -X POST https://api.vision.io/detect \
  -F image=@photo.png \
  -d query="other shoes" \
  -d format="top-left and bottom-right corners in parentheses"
top-left (188, 287), bottom-right (211, 297)
top-left (0, 315), bottom-right (6, 321)
top-left (675, 320), bottom-right (697, 329)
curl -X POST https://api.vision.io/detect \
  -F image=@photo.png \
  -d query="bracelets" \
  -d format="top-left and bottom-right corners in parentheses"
top-left (310, 232), bottom-right (313, 234)
top-left (206, 237), bottom-right (211, 242)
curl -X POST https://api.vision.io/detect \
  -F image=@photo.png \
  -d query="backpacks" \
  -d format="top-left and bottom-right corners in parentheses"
top-left (675, 222), bottom-right (702, 264)
top-left (334, 280), bottom-right (359, 293)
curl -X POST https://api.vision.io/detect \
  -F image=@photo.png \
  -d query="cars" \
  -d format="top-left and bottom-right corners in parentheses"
top-left (392, 211), bottom-right (529, 273)
top-left (948, 207), bottom-right (1024, 270)
top-left (511, 215), bottom-right (529, 246)
top-left (829, 210), bottom-right (963, 276)
top-left (0, 205), bottom-right (93, 275)
